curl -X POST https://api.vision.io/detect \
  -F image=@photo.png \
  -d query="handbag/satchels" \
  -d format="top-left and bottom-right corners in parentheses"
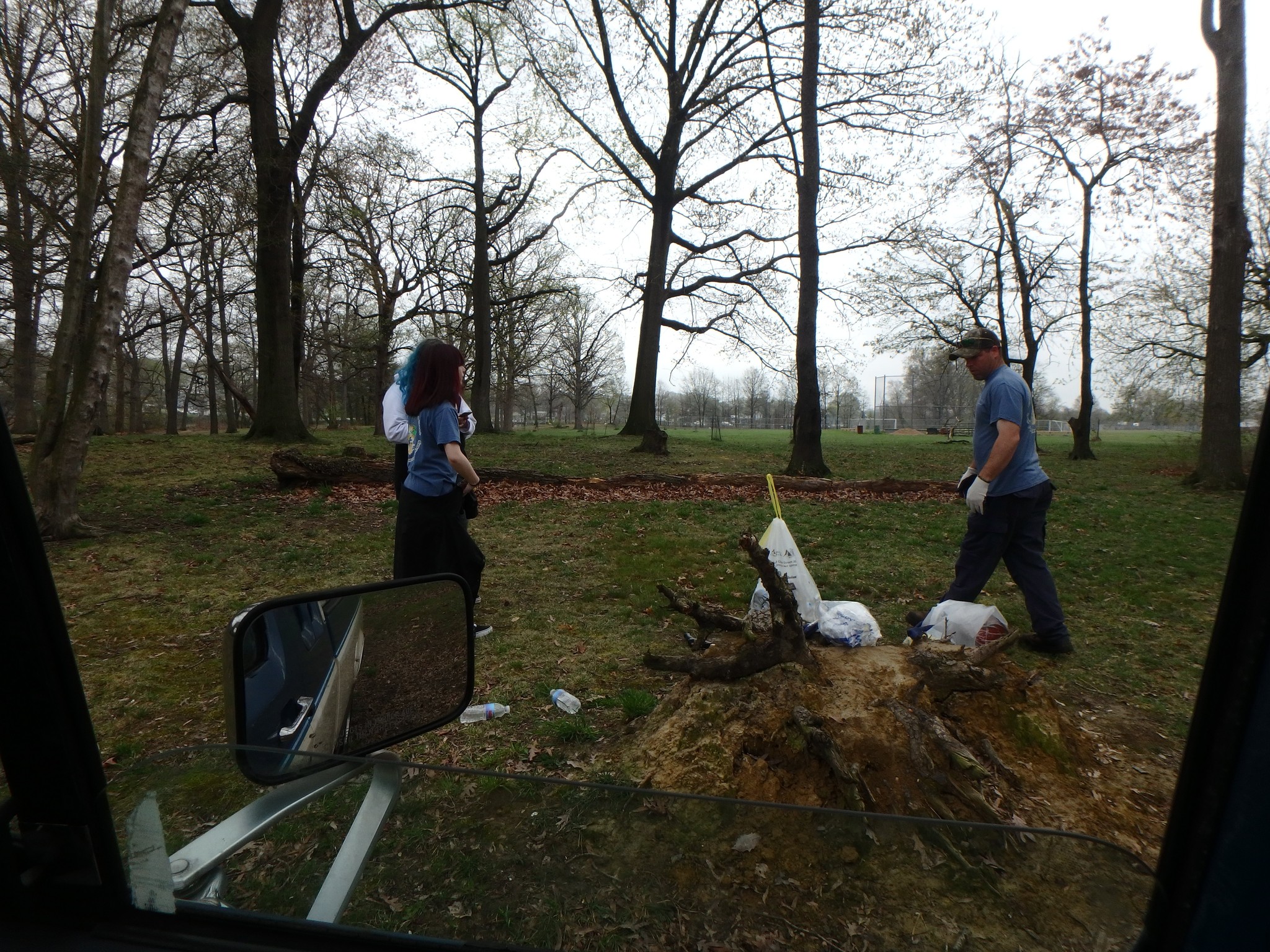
top-left (743, 474), bottom-right (821, 635)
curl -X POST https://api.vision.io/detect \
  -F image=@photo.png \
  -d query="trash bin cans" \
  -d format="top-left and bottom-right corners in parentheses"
top-left (927, 428), bottom-right (937, 434)
top-left (874, 425), bottom-right (880, 435)
top-left (857, 425), bottom-right (863, 434)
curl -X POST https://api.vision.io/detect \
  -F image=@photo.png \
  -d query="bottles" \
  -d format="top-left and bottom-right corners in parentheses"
top-left (550, 688), bottom-right (581, 714)
top-left (460, 703), bottom-right (510, 723)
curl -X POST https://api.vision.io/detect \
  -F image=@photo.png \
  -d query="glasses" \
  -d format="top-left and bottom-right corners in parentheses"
top-left (956, 337), bottom-right (994, 349)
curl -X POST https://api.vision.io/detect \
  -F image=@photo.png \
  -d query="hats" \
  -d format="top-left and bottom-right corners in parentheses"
top-left (949, 327), bottom-right (1003, 360)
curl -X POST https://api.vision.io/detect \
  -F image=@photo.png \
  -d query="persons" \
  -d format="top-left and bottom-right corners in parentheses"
top-left (905, 328), bottom-right (1070, 654)
top-left (382, 339), bottom-right (481, 605)
top-left (402, 344), bottom-right (493, 639)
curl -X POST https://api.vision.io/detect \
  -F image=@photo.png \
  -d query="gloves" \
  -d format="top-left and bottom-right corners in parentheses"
top-left (965, 475), bottom-right (990, 514)
top-left (956, 467), bottom-right (977, 498)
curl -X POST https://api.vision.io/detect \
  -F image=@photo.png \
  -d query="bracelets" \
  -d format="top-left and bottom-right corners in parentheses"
top-left (469, 477), bottom-right (481, 486)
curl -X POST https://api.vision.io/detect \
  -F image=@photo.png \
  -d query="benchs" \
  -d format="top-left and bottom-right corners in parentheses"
top-left (841, 428), bottom-right (856, 432)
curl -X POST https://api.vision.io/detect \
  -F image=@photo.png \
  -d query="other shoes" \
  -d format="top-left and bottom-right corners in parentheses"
top-left (475, 623), bottom-right (492, 637)
top-left (1018, 633), bottom-right (1073, 654)
top-left (475, 595), bottom-right (481, 603)
